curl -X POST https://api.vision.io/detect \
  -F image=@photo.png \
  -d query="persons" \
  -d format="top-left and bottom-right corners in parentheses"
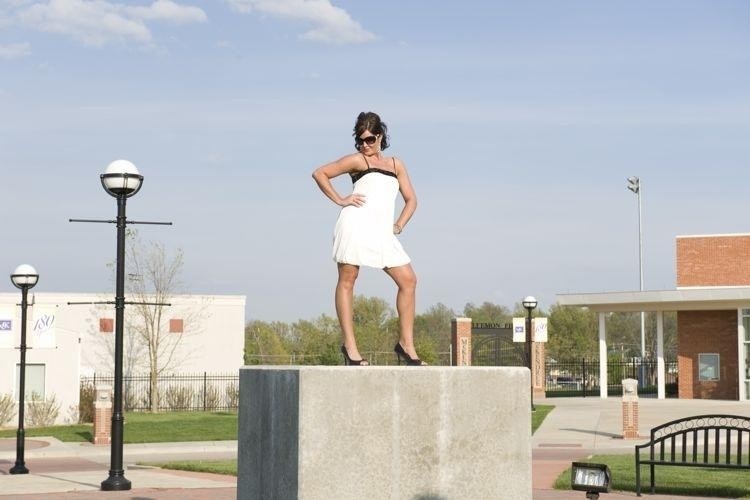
top-left (311, 110), bottom-right (428, 368)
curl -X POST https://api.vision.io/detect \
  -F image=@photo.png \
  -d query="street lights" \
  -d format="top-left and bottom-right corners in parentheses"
top-left (101, 160), bottom-right (144, 478)
top-left (522, 297), bottom-right (537, 407)
top-left (10, 264), bottom-right (40, 464)
top-left (628, 177), bottom-right (645, 387)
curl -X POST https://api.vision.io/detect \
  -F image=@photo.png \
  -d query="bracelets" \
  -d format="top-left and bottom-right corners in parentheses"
top-left (394, 223), bottom-right (404, 235)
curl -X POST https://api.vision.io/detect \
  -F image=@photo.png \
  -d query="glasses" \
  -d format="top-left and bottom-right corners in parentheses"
top-left (355, 136), bottom-right (378, 146)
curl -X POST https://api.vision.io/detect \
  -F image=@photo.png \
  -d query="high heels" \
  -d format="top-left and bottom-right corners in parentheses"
top-left (342, 344), bottom-right (370, 365)
top-left (394, 343), bottom-right (427, 365)
